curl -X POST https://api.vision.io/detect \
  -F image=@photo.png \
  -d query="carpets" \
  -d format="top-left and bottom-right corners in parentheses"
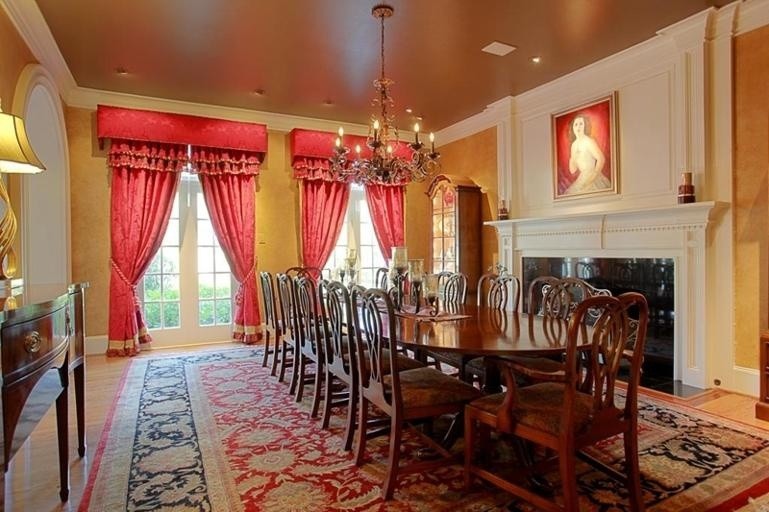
top-left (78, 342), bottom-right (769, 512)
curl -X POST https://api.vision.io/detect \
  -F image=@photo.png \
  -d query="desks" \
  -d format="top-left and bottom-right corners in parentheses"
top-left (0, 279), bottom-right (94, 511)
top-left (311, 292), bottom-right (613, 494)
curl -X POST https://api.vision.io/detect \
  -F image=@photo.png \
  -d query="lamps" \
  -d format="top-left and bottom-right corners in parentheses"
top-left (327, 3), bottom-right (443, 190)
top-left (0, 100), bottom-right (42, 289)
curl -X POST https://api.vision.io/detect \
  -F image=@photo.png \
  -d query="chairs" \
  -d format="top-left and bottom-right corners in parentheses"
top-left (349, 284), bottom-right (481, 503)
top-left (316, 280), bottom-right (426, 452)
top-left (259, 271), bottom-right (319, 377)
top-left (290, 274), bottom-right (375, 419)
top-left (529, 276), bottom-right (613, 327)
top-left (275, 273), bottom-right (339, 394)
top-left (463, 290), bottom-right (657, 510)
top-left (478, 274), bottom-right (523, 308)
top-left (436, 272), bottom-right (469, 303)
top-left (375, 267), bottom-right (409, 294)
top-left (286, 266), bottom-right (324, 283)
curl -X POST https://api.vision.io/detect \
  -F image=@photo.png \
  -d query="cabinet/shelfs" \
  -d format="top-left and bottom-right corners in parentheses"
top-left (419, 171), bottom-right (485, 295)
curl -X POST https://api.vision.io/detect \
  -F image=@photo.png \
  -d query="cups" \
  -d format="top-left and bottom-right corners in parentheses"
top-left (498, 200), bottom-right (506, 208)
top-left (681, 172), bottom-right (692, 184)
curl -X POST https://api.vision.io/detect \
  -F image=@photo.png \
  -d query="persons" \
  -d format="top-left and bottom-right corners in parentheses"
top-left (561, 113), bottom-right (612, 194)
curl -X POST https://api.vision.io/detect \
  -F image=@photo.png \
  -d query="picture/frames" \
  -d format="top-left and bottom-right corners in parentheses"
top-left (548, 87), bottom-right (626, 205)
